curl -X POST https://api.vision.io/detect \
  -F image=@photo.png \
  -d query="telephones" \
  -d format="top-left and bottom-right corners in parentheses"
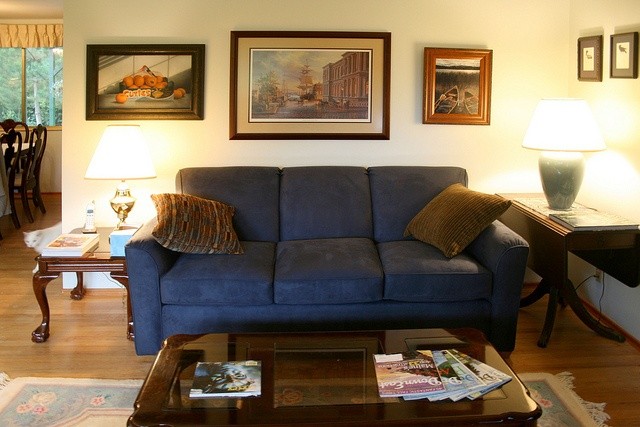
top-left (82, 200), bottom-right (96, 232)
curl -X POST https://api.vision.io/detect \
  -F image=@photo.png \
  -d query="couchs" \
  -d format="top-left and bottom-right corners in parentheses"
top-left (124, 165), bottom-right (531, 356)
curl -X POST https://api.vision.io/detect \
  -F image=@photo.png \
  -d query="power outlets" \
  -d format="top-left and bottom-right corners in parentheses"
top-left (595, 268), bottom-right (604, 283)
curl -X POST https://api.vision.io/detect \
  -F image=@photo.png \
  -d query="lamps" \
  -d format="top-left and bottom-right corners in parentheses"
top-left (82, 123), bottom-right (158, 229)
top-left (522, 96), bottom-right (609, 212)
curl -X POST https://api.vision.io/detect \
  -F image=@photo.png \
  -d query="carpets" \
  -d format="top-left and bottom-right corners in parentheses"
top-left (0, 370), bottom-right (612, 427)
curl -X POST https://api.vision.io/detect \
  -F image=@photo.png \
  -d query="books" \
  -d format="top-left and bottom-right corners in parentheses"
top-left (188, 359), bottom-right (260, 396)
top-left (448, 347), bottom-right (511, 399)
top-left (371, 349), bottom-right (446, 397)
top-left (41, 233), bottom-right (99, 256)
top-left (427, 349), bottom-right (487, 401)
top-left (402, 349), bottom-right (466, 399)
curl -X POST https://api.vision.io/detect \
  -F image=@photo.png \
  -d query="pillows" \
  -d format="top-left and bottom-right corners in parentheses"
top-left (402, 182), bottom-right (513, 260)
top-left (148, 192), bottom-right (245, 256)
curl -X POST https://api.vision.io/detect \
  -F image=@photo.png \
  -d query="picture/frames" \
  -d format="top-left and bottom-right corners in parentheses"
top-left (576, 35), bottom-right (603, 82)
top-left (229, 29), bottom-right (390, 140)
top-left (422, 46), bottom-right (493, 125)
top-left (608, 32), bottom-right (637, 79)
top-left (85, 44), bottom-right (206, 121)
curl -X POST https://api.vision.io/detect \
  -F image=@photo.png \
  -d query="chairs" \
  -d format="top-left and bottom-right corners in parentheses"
top-left (0, 129), bottom-right (23, 240)
top-left (12, 123), bottom-right (48, 224)
top-left (0, 118), bottom-right (30, 193)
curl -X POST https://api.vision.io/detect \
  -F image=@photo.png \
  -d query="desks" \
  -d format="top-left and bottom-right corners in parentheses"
top-left (494, 191), bottom-right (640, 349)
top-left (2, 142), bottom-right (37, 207)
top-left (31, 225), bottom-right (139, 344)
